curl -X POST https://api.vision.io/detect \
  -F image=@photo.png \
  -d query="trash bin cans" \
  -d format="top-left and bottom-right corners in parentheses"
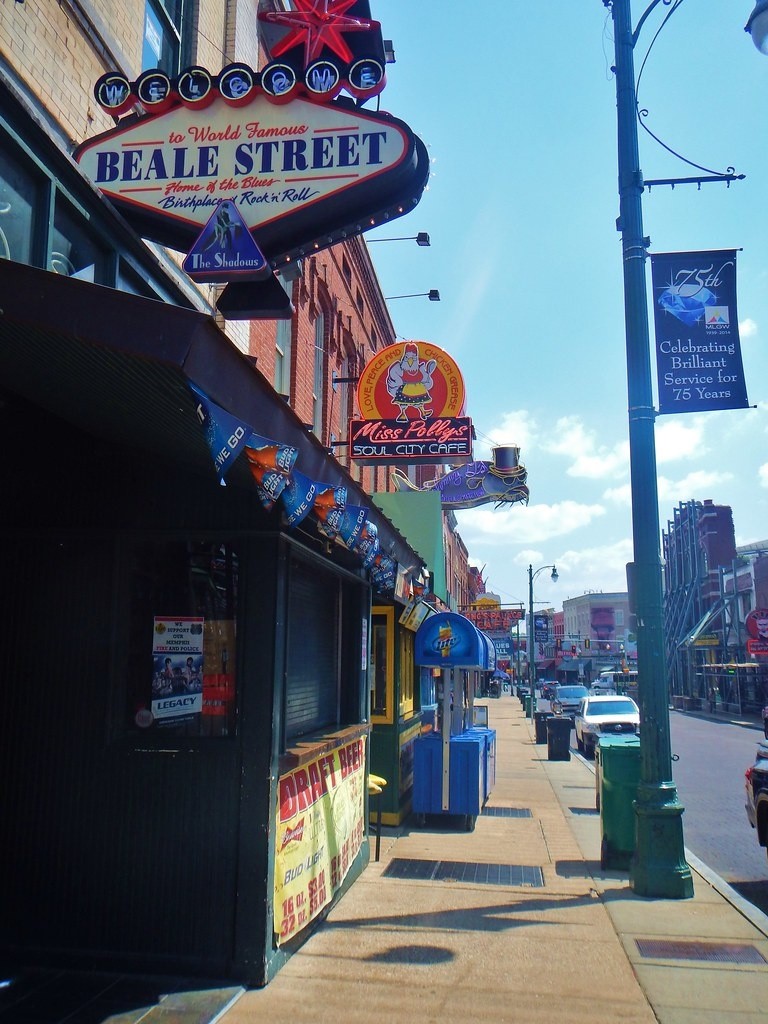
top-left (593, 733), bottom-right (641, 869)
top-left (517, 684), bottom-right (530, 711)
top-left (525, 696), bottom-right (537, 717)
top-left (533, 711), bottom-right (554, 745)
top-left (547, 716), bottom-right (572, 761)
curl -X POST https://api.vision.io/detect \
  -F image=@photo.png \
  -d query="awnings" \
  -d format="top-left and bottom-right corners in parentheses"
top-left (0, 259), bottom-right (426, 593)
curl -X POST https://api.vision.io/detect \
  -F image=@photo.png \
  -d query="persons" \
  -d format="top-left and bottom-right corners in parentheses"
top-left (165, 657), bottom-right (197, 693)
top-left (710, 689), bottom-right (716, 713)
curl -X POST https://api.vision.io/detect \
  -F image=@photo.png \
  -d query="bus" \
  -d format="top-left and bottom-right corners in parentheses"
top-left (591, 670), bottom-right (639, 689)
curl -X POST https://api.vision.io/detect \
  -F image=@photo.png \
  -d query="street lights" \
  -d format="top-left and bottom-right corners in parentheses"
top-left (602, 0), bottom-right (768, 897)
top-left (526, 563), bottom-right (559, 709)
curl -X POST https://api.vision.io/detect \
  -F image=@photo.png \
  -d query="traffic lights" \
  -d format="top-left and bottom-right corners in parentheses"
top-left (572, 645), bottom-right (576, 653)
top-left (585, 639), bottom-right (590, 648)
top-left (556, 639), bottom-right (562, 649)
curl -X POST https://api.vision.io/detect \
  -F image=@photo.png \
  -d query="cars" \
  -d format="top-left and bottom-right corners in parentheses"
top-left (550, 684), bottom-right (589, 717)
top-left (534, 680), bottom-right (562, 699)
top-left (574, 696), bottom-right (640, 758)
